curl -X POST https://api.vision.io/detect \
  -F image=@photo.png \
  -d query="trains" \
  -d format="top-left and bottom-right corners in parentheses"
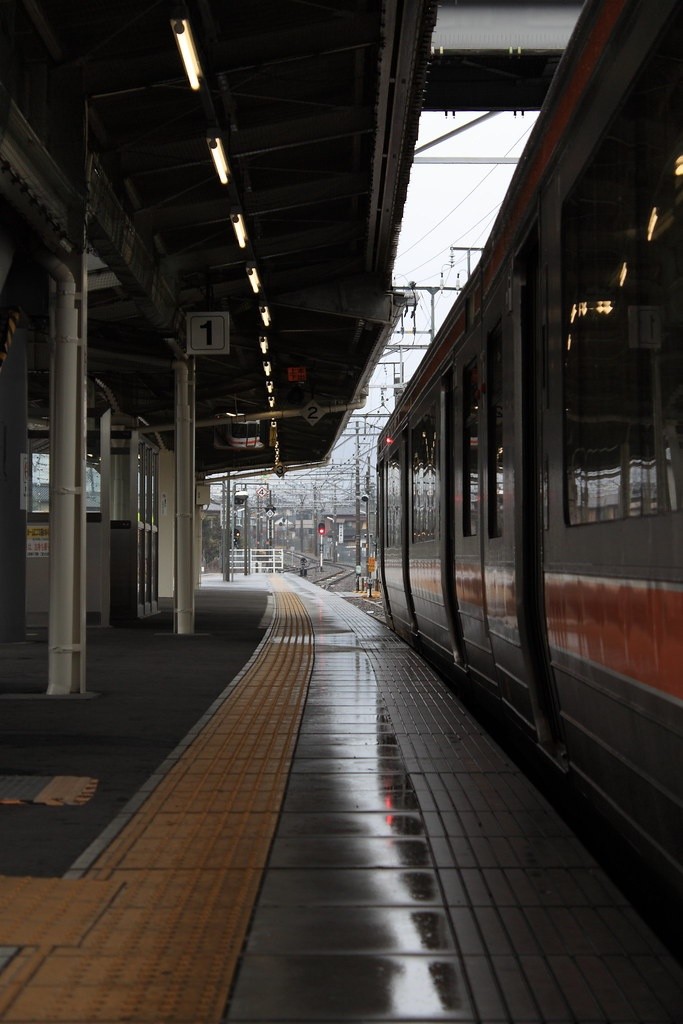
top-left (376, 1), bottom-right (683, 923)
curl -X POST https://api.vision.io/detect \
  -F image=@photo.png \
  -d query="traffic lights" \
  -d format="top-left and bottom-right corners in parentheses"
top-left (318, 522), bottom-right (325, 535)
